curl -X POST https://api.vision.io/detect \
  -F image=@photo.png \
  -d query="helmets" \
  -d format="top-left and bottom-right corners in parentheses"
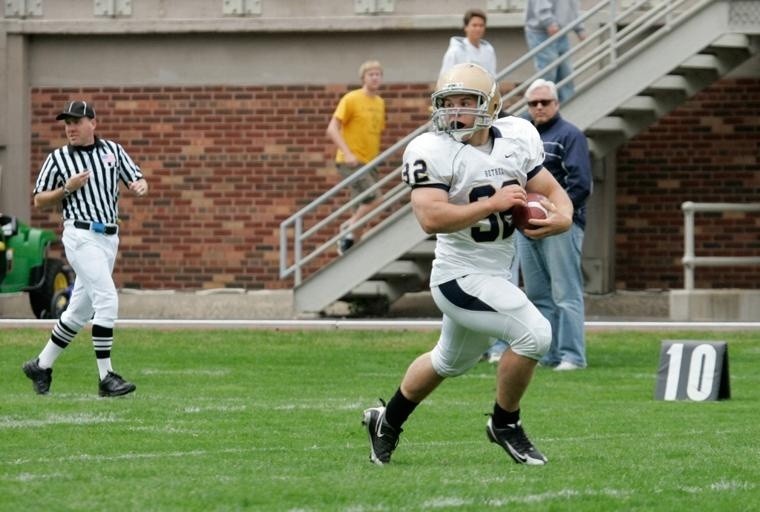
top-left (430, 62), bottom-right (503, 144)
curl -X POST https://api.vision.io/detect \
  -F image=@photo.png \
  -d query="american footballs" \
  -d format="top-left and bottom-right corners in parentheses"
top-left (511, 193), bottom-right (549, 241)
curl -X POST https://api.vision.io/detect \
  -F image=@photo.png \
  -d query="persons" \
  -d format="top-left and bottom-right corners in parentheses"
top-left (479, 248), bottom-right (521, 362)
top-left (520, 0), bottom-right (592, 103)
top-left (514, 78), bottom-right (594, 373)
top-left (431, 7), bottom-right (499, 118)
top-left (324, 57), bottom-right (388, 259)
top-left (20, 97), bottom-right (149, 400)
top-left (359, 61), bottom-right (575, 468)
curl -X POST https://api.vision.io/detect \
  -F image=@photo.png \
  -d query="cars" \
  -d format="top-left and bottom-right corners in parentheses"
top-left (0, 213), bottom-right (74, 318)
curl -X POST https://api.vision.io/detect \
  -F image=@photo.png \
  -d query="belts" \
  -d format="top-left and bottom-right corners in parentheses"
top-left (73, 220), bottom-right (119, 235)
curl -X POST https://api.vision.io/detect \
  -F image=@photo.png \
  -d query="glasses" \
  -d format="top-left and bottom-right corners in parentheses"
top-left (526, 97), bottom-right (556, 108)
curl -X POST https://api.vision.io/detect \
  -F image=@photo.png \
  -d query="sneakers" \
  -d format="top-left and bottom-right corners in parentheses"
top-left (487, 352), bottom-right (504, 364)
top-left (22, 356), bottom-right (55, 395)
top-left (552, 358), bottom-right (584, 372)
top-left (361, 406), bottom-right (404, 467)
top-left (96, 371), bottom-right (137, 398)
top-left (485, 413), bottom-right (551, 467)
top-left (336, 221), bottom-right (357, 257)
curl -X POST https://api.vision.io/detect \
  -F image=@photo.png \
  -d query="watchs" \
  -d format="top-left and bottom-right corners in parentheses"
top-left (63, 185), bottom-right (70, 197)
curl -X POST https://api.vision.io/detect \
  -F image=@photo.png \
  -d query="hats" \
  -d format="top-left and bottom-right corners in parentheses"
top-left (55, 100), bottom-right (97, 121)
